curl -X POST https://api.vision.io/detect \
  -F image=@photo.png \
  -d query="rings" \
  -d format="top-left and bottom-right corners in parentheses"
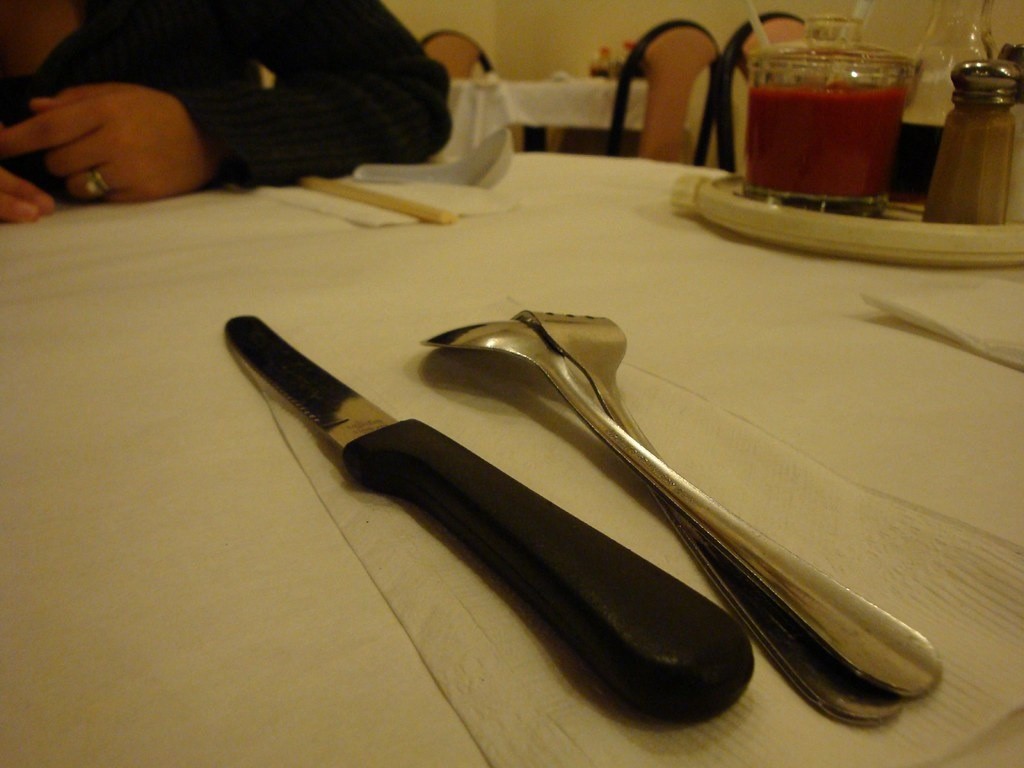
top-left (85, 168), bottom-right (110, 196)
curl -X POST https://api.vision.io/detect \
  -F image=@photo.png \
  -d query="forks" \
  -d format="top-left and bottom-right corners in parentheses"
top-left (512, 308), bottom-right (911, 725)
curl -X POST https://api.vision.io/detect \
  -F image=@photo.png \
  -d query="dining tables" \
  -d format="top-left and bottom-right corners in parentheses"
top-left (443, 78), bottom-right (723, 167)
top-left (0, 154), bottom-right (1023, 767)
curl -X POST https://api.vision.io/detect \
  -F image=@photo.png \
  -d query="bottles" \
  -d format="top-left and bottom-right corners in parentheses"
top-left (923, 45), bottom-right (1024, 225)
top-left (587, 37), bottom-right (641, 79)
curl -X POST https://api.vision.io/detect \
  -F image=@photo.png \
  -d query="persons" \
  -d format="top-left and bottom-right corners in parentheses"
top-left (0, 0), bottom-right (451, 223)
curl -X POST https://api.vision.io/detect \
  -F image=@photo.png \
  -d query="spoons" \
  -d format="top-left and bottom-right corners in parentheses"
top-left (417, 320), bottom-right (942, 700)
top-left (355, 129), bottom-right (515, 188)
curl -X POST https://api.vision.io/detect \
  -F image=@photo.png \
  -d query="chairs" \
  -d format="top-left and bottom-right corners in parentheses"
top-left (715, 11), bottom-right (805, 173)
top-left (422, 29), bottom-right (493, 79)
top-left (608, 19), bottom-right (721, 165)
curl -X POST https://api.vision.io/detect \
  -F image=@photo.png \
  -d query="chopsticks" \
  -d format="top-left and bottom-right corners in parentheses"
top-left (300, 171), bottom-right (458, 226)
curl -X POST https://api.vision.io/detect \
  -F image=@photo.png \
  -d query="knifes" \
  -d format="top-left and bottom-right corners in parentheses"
top-left (224, 311), bottom-right (755, 730)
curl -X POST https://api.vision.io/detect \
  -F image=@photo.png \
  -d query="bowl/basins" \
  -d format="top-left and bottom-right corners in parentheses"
top-left (742, 12), bottom-right (919, 213)
